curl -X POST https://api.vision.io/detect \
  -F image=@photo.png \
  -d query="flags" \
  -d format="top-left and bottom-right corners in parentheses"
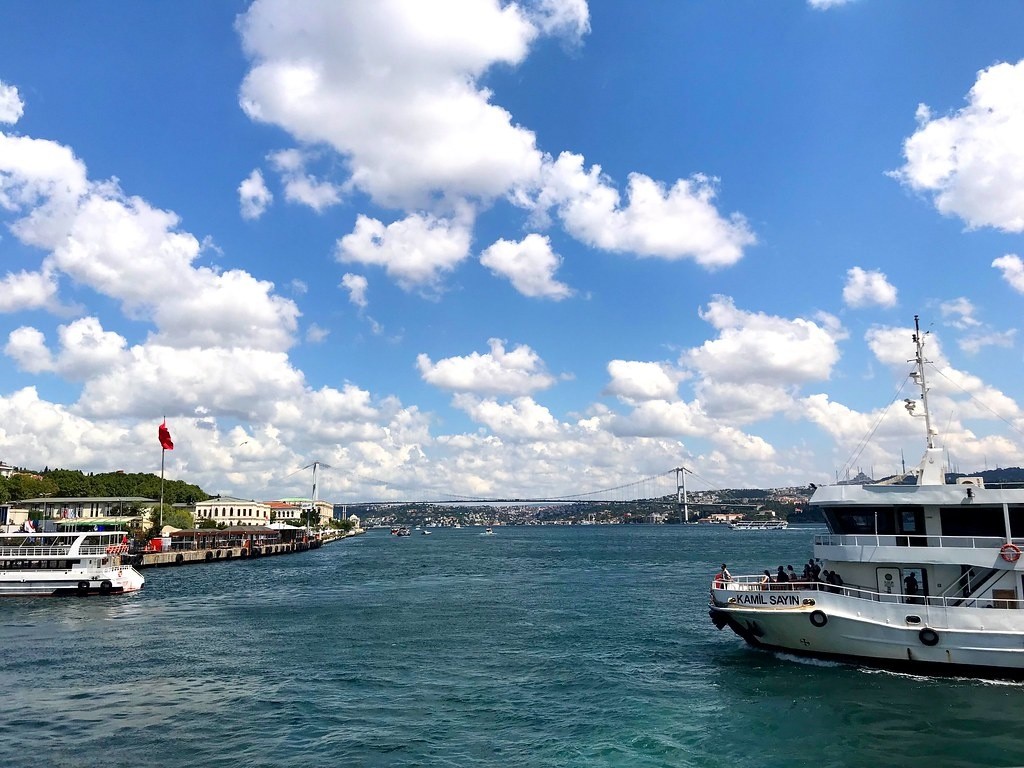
top-left (159, 423), bottom-right (173, 450)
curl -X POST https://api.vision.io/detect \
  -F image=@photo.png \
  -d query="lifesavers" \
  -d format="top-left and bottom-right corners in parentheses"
top-left (105, 544), bottom-right (130, 553)
top-left (118, 570), bottom-right (123, 577)
top-left (997, 542), bottom-right (1021, 562)
top-left (919, 627), bottom-right (940, 647)
top-left (809, 610), bottom-right (828, 627)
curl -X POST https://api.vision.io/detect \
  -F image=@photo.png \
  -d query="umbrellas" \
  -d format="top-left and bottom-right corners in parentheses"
top-left (265, 523), bottom-right (301, 530)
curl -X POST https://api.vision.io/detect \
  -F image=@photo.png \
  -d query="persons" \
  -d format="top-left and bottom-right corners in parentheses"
top-left (720, 564), bottom-right (730, 590)
top-left (759, 559), bottom-right (843, 594)
top-left (904, 572), bottom-right (918, 595)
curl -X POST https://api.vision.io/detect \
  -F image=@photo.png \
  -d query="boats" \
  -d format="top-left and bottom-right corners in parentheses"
top-left (423, 530), bottom-right (432, 536)
top-left (0, 532), bottom-right (145, 596)
top-left (486, 527), bottom-right (492, 534)
top-left (729, 520), bottom-right (788, 531)
top-left (392, 524), bottom-right (410, 535)
top-left (710, 483), bottom-right (1024, 671)
